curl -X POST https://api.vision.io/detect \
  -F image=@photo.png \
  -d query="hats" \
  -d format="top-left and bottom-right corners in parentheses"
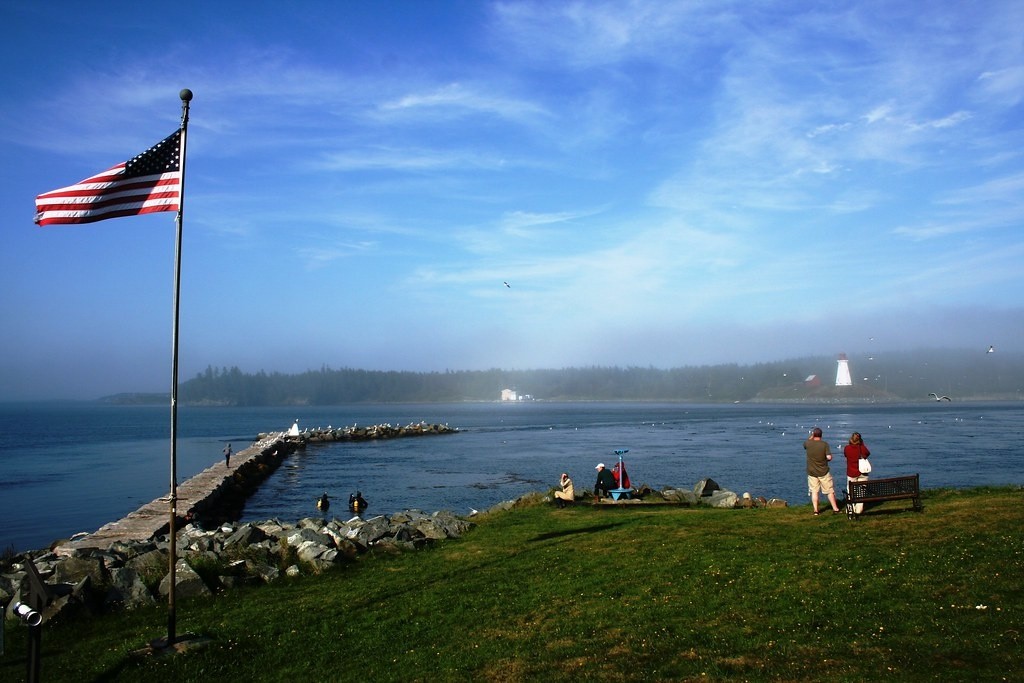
top-left (595, 463), bottom-right (605, 468)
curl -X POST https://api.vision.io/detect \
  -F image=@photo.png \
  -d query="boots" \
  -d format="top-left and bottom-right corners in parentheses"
top-left (556, 498), bottom-right (563, 509)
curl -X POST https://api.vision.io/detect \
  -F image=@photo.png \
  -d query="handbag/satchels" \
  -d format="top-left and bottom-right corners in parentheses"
top-left (858, 445), bottom-right (871, 473)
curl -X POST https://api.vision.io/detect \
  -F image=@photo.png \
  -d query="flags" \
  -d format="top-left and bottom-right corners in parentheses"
top-left (34, 127), bottom-right (181, 227)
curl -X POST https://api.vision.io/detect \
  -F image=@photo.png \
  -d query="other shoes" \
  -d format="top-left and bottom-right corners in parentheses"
top-left (833, 509), bottom-right (846, 515)
top-left (592, 496), bottom-right (599, 503)
top-left (814, 511), bottom-right (822, 515)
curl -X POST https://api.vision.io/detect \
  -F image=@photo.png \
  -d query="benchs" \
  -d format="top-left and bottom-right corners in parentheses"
top-left (841, 472), bottom-right (924, 521)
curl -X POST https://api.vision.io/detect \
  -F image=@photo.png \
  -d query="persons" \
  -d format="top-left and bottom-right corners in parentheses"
top-left (222, 443), bottom-right (233, 468)
top-left (554, 472), bottom-right (574, 508)
top-left (802, 427), bottom-right (841, 516)
top-left (349, 491), bottom-right (368, 508)
top-left (592, 463), bottom-right (618, 503)
top-left (318, 493), bottom-right (329, 508)
top-left (843, 431), bottom-right (871, 518)
top-left (611, 461), bottom-right (630, 489)
